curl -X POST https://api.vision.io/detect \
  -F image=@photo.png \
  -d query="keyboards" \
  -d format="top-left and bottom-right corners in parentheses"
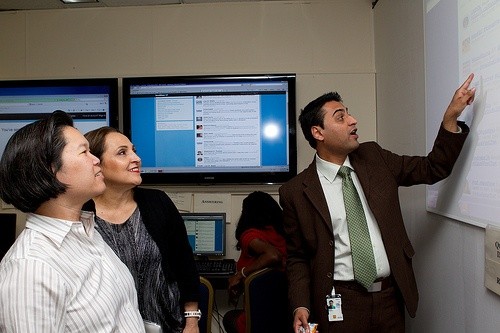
top-left (194, 259), bottom-right (235, 275)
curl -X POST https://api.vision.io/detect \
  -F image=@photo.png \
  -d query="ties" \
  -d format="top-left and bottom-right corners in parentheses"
top-left (337, 166), bottom-right (377, 289)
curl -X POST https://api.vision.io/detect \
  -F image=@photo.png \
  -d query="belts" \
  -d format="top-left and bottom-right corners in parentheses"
top-left (333, 277), bottom-right (394, 292)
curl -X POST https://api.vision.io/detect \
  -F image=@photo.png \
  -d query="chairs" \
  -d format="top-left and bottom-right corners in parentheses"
top-left (246, 265), bottom-right (293, 333)
top-left (198, 274), bottom-right (214, 333)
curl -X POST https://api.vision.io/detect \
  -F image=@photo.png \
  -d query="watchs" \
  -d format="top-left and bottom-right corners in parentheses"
top-left (184, 309), bottom-right (201, 320)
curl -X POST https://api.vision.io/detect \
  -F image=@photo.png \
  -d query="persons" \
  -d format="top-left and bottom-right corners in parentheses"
top-left (80, 127), bottom-right (206, 333)
top-left (329, 300), bottom-right (335, 309)
top-left (223, 190), bottom-right (300, 333)
top-left (0, 110), bottom-right (146, 333)
top-left (197, 151), bottom-right (203, 155)
top-left (279, 73), bottom-right (476, 333)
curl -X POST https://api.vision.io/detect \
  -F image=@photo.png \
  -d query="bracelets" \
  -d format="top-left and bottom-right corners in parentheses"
top-left (241, 267), bottom-right (246, 278)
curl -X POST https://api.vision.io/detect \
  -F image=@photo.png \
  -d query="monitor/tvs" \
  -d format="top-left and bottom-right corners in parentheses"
top-left (122, 73), bottom-right (297, 186)
top-left (179, 212), bottom-right (226, 257)
top-left (0, 77), bottom-right (119, 162)
